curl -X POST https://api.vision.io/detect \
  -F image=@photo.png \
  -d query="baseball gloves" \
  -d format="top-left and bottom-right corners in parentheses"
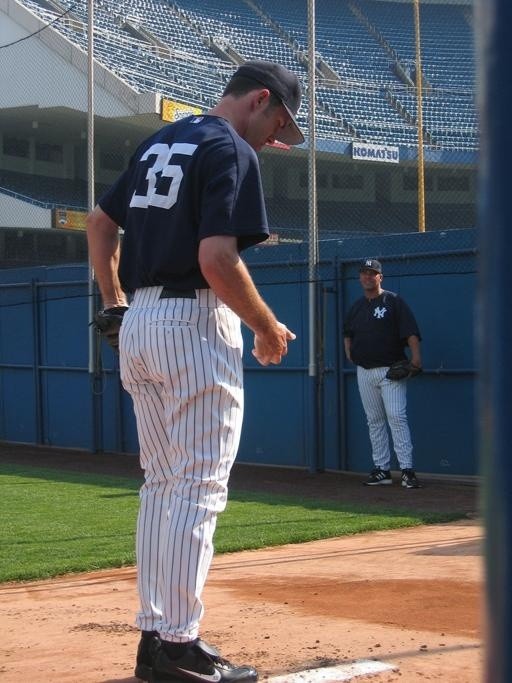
top-left (386, 360), bottom-right (423, 381)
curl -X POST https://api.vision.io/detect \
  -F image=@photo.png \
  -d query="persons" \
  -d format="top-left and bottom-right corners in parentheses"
top-left (344, 258), bottom-right (423, 488)
top-left (85, 59), bottom-right (306, 682)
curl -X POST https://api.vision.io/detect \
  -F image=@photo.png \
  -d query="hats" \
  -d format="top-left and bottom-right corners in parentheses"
top-left (359, 259), bottom-right (382, 274)
top-left (233, 60), bottom-right (305, 145)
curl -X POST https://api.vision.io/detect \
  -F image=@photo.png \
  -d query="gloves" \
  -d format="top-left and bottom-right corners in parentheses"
top-left (392, 363), bottom-right (422, 383)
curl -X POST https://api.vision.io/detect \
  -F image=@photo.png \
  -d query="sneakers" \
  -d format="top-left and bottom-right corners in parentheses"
top-left (401, 469), bottom-right (421, 489)
top-left (135, 630), bottom-right (259, 683)
top-left (362, 471), bottom-right (393, 486)
